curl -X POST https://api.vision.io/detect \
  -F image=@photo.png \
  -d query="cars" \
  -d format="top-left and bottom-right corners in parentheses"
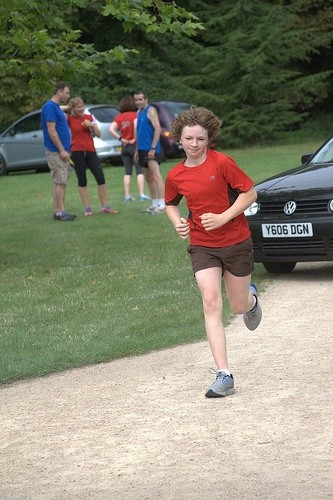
top-left (148, 100), bottom-right (197, 158)
top-left (237, 135), bottom-right (333, 275)
top-left (0, 103), bottom-right (125, 176)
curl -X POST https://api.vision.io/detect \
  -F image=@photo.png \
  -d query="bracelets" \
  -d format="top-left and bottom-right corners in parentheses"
top-left (151, 146), bottom-right (155, 150)
top-left (119, 136), bottom-right (124, 141)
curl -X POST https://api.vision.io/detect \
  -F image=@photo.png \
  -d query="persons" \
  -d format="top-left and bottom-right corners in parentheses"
top-left (109, 95), bottom-right (151, 201)
top-left (134, 90), bottom-right (165, 214)
top-left (59, 97), bottom-right (120, 216)
top-left (163, 105), bottom-right (262, 398)
top-left (41, 83), bottom-right (77, 221)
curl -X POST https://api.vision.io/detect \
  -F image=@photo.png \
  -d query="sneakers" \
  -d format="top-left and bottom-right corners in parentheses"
top-left (243, 284), bottom-right (262, 331)
top-left (204, 367), bottom-right (235, 397)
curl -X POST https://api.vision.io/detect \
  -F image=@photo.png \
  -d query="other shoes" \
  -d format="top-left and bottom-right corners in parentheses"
top-left (83, 208), bottom-right (93, 217)
top-left (141, 203), bottom-right (165, 216)
top-left (54, 210), bottom-right (77, 221)
top-left (139, 194), bottom-right (152, 201)
top-left (124, 196), bottom-right (136, 202)
top-left (100, 205), bottom-right (120, 214)
